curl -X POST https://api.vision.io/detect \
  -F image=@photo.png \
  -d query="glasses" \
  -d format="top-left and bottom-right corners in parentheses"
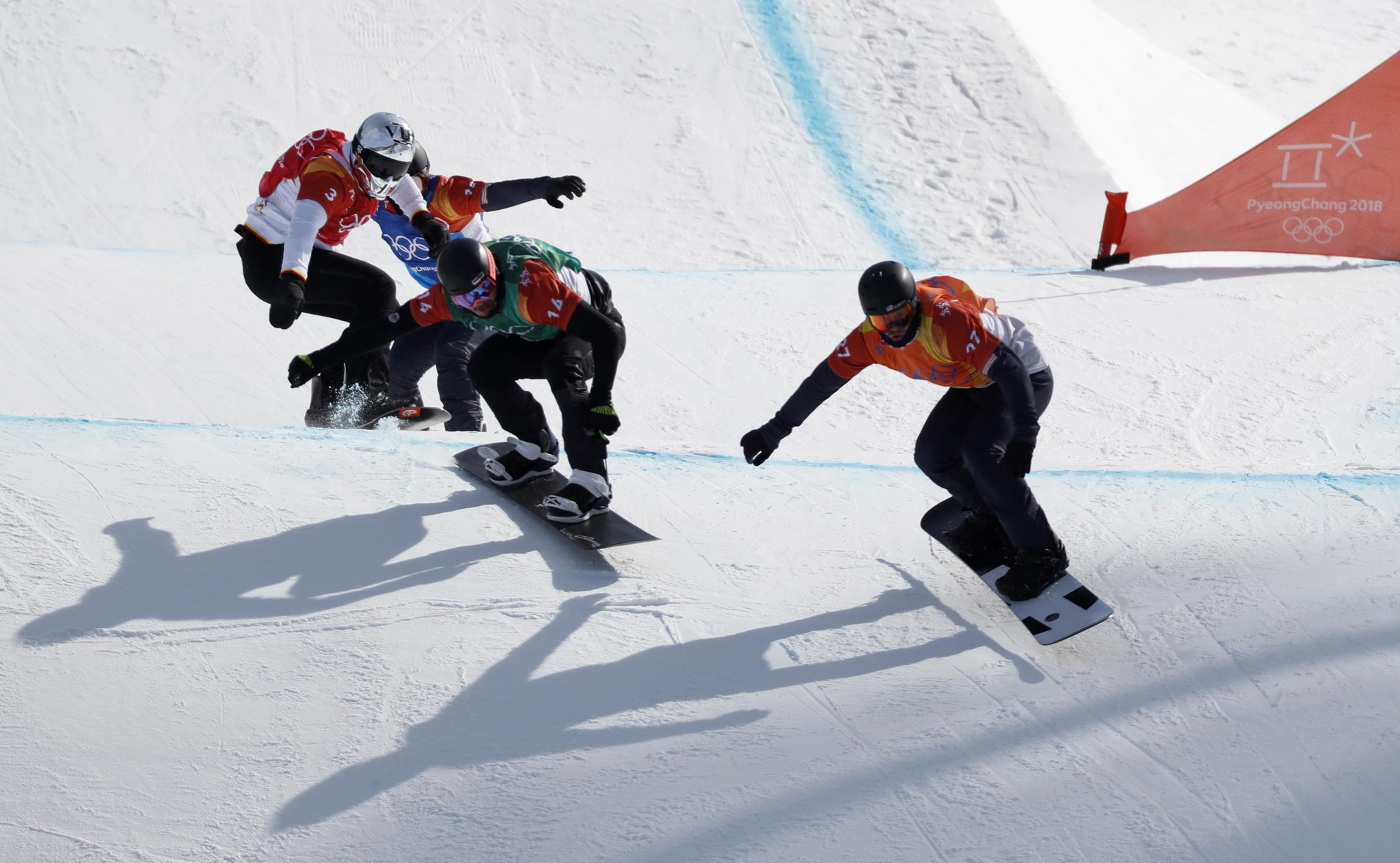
top-left (868, 303), bottom-right (915, 332)
top-left (451, 277), bottom-right (496, 309)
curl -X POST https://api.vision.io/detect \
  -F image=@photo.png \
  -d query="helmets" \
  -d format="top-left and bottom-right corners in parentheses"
top-left (858, 260), bottom-right (923, 348)
top-left (349, 111), bottom-right (417, 201)
top-left (436, 237), bottom-right (506, 320)
top-left (407, 139), bottom-right (430, 177)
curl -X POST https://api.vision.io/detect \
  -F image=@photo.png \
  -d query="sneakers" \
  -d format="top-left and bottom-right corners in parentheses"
top-left (995, 538), bottom-right (1069, 602)
top-left (948, 510), bottom-right (1011, 550)
top-left (486, 429), bottom-right (553, 481)
top-left (544, 456), bottom-right (612, 516)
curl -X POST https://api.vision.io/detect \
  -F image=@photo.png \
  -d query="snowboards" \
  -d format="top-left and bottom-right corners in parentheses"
top-left (453, 442), bottom-right (663, 552)
top-left (357, 404), bottom-right (454, 432)
top-left (920, 494), bottom-right (1114, 647)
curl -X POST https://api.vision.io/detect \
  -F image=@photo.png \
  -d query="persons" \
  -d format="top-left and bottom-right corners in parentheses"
top-left (371, 140), bottom-right (586, 432)
top-left (285, 236), bottom-right (627, 522)
top-left (739, 258), bottom-right (1070, 603)
top-left (235, 112), bottom-right (416, 427)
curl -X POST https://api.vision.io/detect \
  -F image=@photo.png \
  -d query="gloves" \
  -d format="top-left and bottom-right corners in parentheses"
top-left (415, 212), bottom-right (452, 260)
top-left (287, 354), bottom-right (319, 389)
top-left (545, 175), bottom-right (587, 210)
top-left (740, 428), bottom-right (776, 467)
top-left (269, 272), bottom-right (306, 329)
top-left (584, 400), bottom-right (621, 444)
top-left (1003, 439), bottom-right (1041, 481)
